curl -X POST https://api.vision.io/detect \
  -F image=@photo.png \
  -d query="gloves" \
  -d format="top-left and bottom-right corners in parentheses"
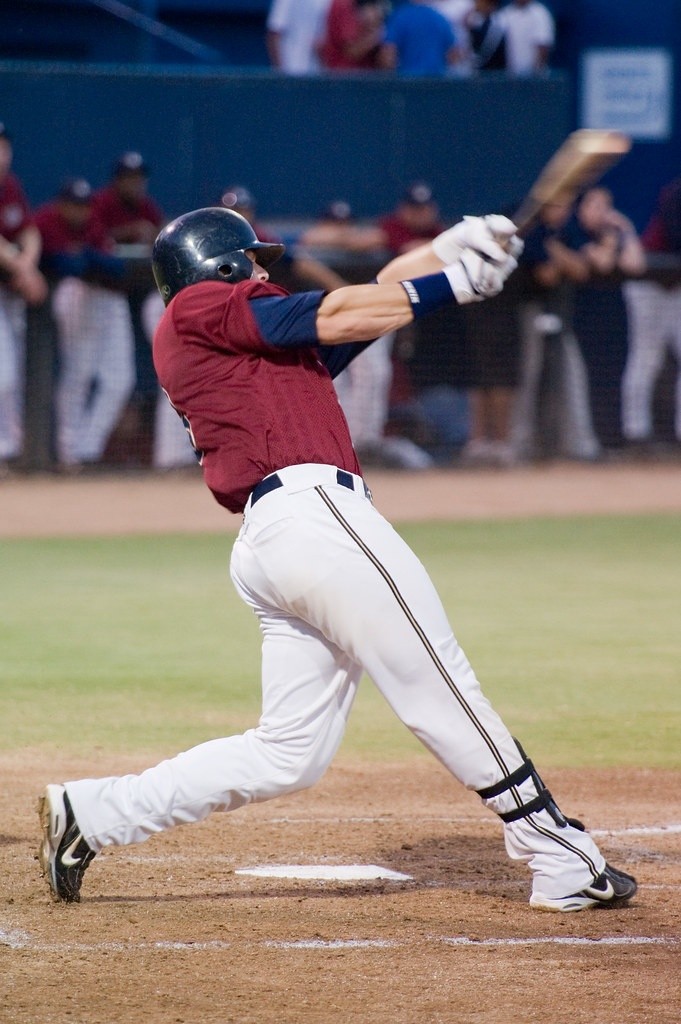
top-left (442, 236), bottom-right (525, 304)
top-left (432, 213), bottom-right (523, 265)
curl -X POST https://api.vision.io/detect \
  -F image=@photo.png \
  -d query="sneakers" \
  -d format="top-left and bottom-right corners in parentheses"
top-left (34, 783), bottom-right (96, 904)
top-left (530, 861), bottom-right (638, 913)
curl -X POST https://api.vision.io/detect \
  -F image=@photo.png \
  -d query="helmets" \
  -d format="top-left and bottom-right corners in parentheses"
top-left (150, 206), bottom-right (284, 309)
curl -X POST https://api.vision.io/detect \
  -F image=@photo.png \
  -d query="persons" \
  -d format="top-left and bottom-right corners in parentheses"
top-left (505, 186), bottom-right (681, 460)
top-left (266, 0), bottom-right (557, 80)
top-left (35, 208), bottom-right (638, 913)
top-left (0, 122), bottom-right (502, 464)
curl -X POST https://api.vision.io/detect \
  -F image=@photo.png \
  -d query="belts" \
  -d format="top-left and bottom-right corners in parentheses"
top-left (250, 468), bottom-right (373, 507)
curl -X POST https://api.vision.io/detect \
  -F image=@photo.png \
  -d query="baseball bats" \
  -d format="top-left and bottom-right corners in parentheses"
top-left (481, 126), bottom-right (632, 264)
